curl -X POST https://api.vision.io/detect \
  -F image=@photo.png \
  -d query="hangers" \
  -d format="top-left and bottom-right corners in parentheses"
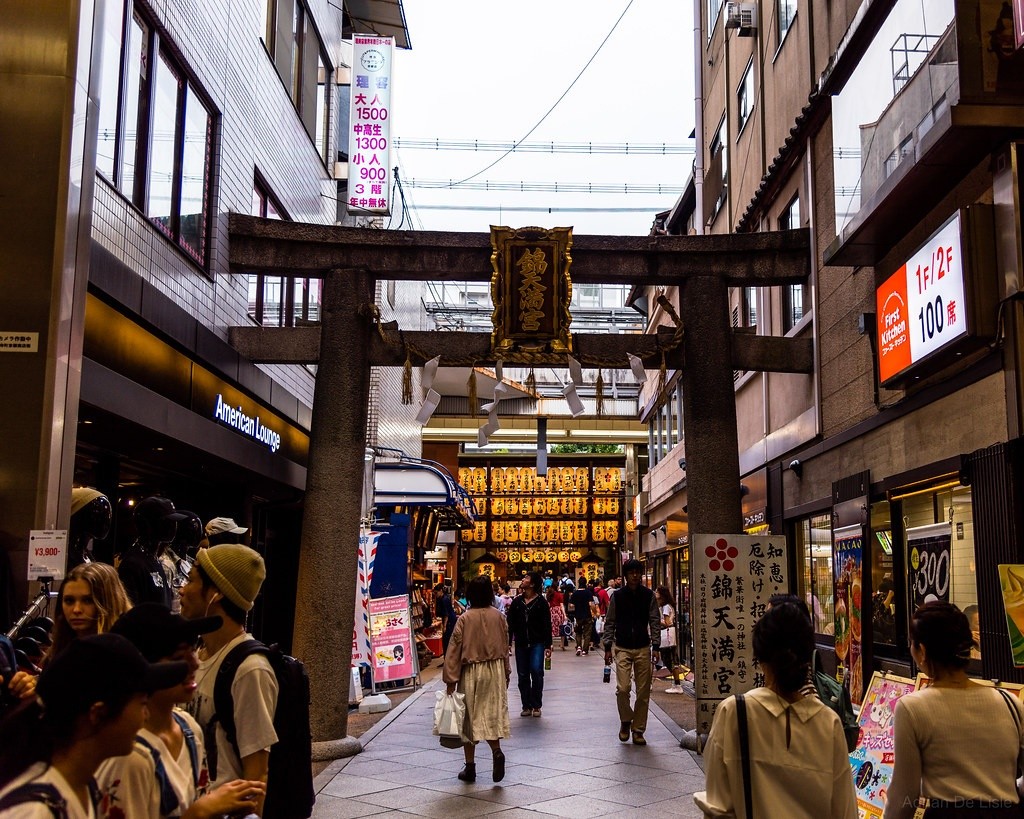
top-left (0, 585), bottom-right (55, 704)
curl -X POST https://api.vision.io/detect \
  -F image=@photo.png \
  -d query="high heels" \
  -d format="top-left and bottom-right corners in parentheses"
top-left (551, 645), bottom-right (554, 650)
top-left (559, 646), bottom-right (566, 652)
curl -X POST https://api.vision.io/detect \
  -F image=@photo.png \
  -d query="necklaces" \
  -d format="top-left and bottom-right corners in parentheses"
top-left (192, 630), bottom-right (246, 693)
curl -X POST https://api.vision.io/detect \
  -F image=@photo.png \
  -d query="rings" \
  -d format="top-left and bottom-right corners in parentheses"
top-left (32, 680), bottom-right (38, 686)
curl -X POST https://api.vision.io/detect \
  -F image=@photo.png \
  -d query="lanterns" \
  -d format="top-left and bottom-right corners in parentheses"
top-left (457, 465), bottom-right (635, 543)
top-left (478, 562), bottom-right (495, 582)
top-left (496, 551), bottom-right (582, 562)
top-left (583, 562), bottom-right (598, 585)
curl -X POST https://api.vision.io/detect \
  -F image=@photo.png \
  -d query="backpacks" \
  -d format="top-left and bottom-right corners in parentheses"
top-left (813, 667), bottom-right (860, 753)
top-left (214, 640), bottom-right (315, 819)
top-left (561, 578), bottom-right (568, 589)
top-left (593, 589), bottom-right (601, 608)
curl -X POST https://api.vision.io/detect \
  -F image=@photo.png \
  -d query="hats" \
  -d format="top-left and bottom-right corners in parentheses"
top-left (36, 633), bottom-right (189, 710)
top-left (197, 544), bottom-right (266, 611)
top-left (107, 602), bottom-right (224, 663)
top-left (70, 486), bottom-right (107, 517)
top-left (133, 496), bottom-right (187, 534)
top-left (204, 517), bottom-right (249, 536)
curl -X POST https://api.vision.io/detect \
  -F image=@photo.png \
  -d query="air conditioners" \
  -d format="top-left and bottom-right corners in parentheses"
top-left (736, 3), bottom-right (758, 37)
top-left (723, 2), bottom-right (740, 30)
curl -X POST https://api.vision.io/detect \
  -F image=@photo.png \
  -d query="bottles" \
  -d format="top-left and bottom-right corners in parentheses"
top-left (545, 653), bottom-right (551, 670)
top-left (603, 662), bottom-right (611, 683)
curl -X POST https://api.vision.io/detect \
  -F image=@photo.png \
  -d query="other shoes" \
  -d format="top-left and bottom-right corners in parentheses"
top-left (458, 763), bottom-right (476, 782)
top-left (492, 748), bottom-right (505, 782)
top-left (437, 662), bottom-right (444, 669)
top-left (582, 653), bottom-right (589, 657)
top-left (575, 647), bottom-right (581, 656)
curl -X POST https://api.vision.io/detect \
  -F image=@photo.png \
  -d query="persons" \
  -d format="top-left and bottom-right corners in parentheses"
top-left (693, 594), bottom-right (860, 819)
top-left (68, 487), bottom-right (249, 615)
top-left (807, 580), bottom-right (834, 635)
top-left (962, 605), bottom-right (981, 660)
top-left (433, 562), bottom-right (624, 668)
top-left (443, 575), bottom-right (512, 782)
top-left (873, 578), bottom-right (896, 644)
top-left (648, 586), bottom-right (685, 694)
top-left (507, 571), bottom-right (552, 716)
top-left (883, 600), bottom-right (1024, 819)
top-left (603, 558), bottom-right (661, 746)
top-left (0, 543), bottom-right (315, 819)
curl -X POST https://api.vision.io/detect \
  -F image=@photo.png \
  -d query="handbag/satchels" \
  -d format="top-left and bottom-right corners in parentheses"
top-left (559, 622), bottom-right (573, 636)
top-left (431, 690), bottom-right (465, 739)
top-left (439, 737), bottom-right (463, 749)
top-left (659, 627), bottom-right (677, 648)
top-left (595, 617), bottom-right (605, 634)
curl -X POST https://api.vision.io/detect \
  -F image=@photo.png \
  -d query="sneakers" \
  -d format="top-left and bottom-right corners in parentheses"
top-left (619, 721), bottom-right (630, 741)
top-left (665, 685), bottom-right (684, 694)
top-left (521, 709), bottom-right (532, 716)
top-left (533, 708), bottom-right (541, 716)
top-left (632, 733), bottom-right (647, 746)
